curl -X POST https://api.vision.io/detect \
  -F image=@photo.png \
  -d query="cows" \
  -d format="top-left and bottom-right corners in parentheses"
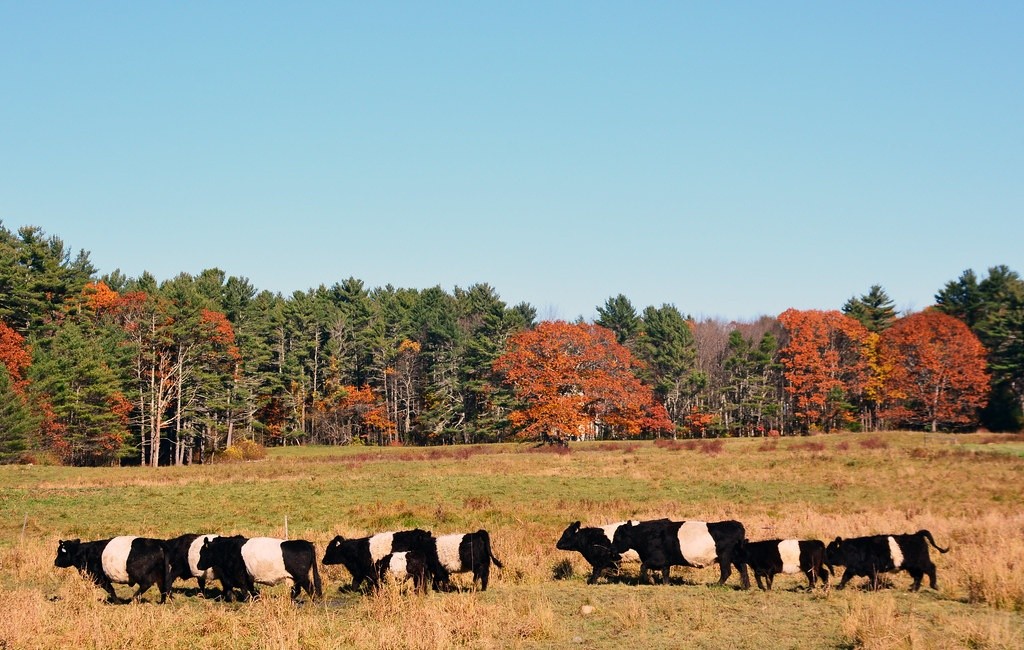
top-left (54, 529), bottom-right (504, 607)
top-left (556, 518), bottom-right (951, 595)
